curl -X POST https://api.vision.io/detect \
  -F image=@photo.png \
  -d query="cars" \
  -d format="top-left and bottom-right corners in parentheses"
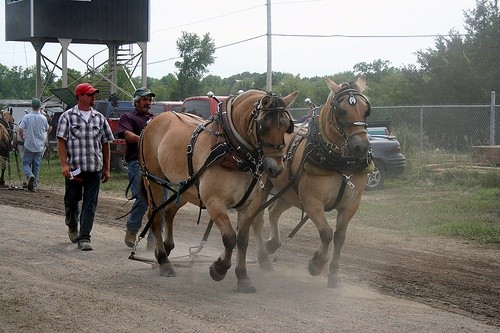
top-left (365, 120), bottom-right (406, 190)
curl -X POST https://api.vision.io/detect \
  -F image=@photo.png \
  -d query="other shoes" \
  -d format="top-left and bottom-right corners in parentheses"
top-left (68, 227), bottom-right (79, 243)
top-left (145, 237), bottom-right (156, 251)
top-left (77, 238), bottom-right (93, 250)
top-left (124, 228), bottom-right (136, 247)
top-left (27, 174), bottom-right (36, 192)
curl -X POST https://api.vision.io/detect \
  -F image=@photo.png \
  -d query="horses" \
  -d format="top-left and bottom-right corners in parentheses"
top-left (0, 107), bottom-right (14, 187)
top-left (264, 74), bottom-right (371, 287)
top-left (139, 90), bottom-right (298, 294)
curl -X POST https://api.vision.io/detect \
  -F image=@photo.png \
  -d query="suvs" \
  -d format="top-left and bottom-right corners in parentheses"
top-left (180, 95), bottom-right (231, 119)
top-left (46, 88), bottom-right (186, 162)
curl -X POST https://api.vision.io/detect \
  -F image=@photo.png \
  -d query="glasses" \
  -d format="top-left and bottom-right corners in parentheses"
top-left (83, 93), bottom-right (96, 97)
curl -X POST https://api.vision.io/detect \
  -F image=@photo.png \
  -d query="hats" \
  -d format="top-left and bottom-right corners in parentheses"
top-left (133, 87), bottom-right (156, 99)
top-left (31, 98), bottom-right (40, 107)
top-left (74, 83), bottom-right (99, 96)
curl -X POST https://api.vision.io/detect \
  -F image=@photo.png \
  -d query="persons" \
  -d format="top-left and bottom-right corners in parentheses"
top-left (118, 87), bottom-right (169, 249)
top-left (19, 98), bottom-right (49, 192)
top-left (56, 84), bottom-right (114, 250)
